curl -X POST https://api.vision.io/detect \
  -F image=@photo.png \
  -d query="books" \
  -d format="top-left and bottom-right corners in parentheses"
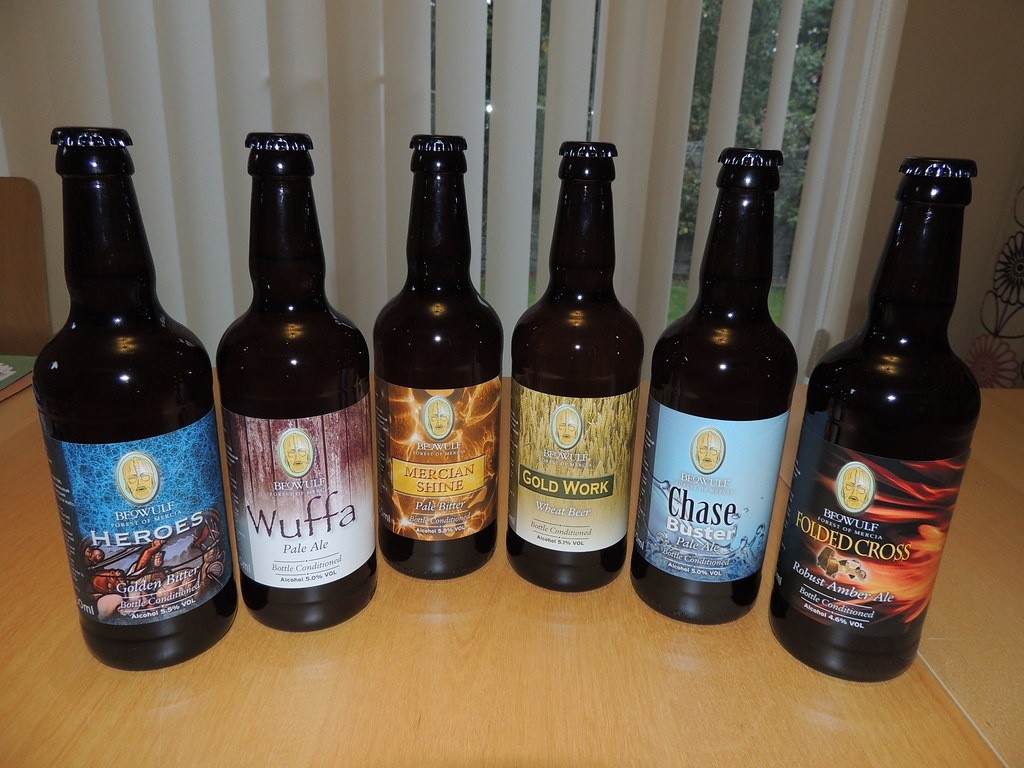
top-left (0, 355), bottom-right (38, 402)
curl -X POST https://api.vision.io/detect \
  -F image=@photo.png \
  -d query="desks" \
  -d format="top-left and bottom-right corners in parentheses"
top-left (1, 385), bottom-right (1024, 768)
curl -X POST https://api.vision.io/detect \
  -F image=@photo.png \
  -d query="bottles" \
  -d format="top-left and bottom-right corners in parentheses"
top-left (371, 133), bottom-right (507, 580)
top-left (503, 141), bottom-right (645, 592)
top-left (214, 132), bottom-right (383, 632)
top-left (629, 144), bottom-right (798, 630)
top-left (767, 155), bottom-right (983, 683)
top-left (31, 127), bottom-right (239, 672)
